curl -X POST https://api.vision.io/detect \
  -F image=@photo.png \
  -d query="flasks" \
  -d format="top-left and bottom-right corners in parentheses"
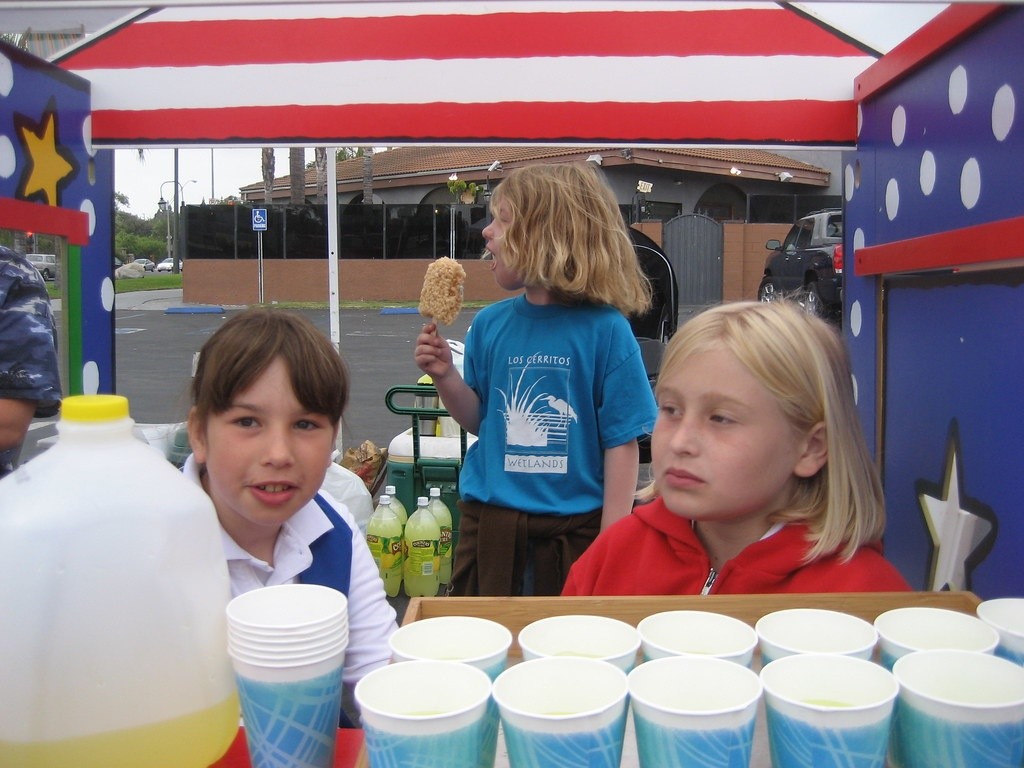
top-left (415, 382), bottom-right (439, 437)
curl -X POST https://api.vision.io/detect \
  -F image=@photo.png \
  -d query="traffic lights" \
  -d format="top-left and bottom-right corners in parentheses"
top-left (29, 233), bottom-right (32, 238)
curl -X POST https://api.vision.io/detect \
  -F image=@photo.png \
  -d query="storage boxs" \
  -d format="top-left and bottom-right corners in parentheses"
top-left (349, 585), bottom-right (995, 768)
top-left (385, 424), bottom-right (480, 548)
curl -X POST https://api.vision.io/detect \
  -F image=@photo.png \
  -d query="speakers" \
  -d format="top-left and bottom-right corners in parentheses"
top-left (778, 171), bottom-right (794, 182)
top-left (586, 154), bottom-right (603, 166)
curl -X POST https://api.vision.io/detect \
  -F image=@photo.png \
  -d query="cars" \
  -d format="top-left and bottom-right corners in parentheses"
top-left (26, 254), bottom-right (55, 280)
top-left (465, 218), bottom-right (488, 253)
top-left (134, 259), bottom-right (156, 272)
top-left (758, 208), bottom-right (842, 320)
top-left (343, 233), bottom-right (384, 259)
top-left (157, 258), bottom-right (184, 272)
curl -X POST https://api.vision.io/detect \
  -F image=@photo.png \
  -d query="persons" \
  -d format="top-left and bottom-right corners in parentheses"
top-left (175, 306), bottom-right (400, 728)
top-left (414, 162), bottom-right (658, 597)
top-left (557, 302), bottom-right (913, 596)
top-left (0, 246), bottom-right (63, 479)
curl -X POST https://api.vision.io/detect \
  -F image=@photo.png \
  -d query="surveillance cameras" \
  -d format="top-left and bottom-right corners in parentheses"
top-left (489, 161), bottom-right (500, 173)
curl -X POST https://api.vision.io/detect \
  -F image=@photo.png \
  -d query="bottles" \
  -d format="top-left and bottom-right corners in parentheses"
top-left (0, 396), bottom-right (238, 767)
top-left (384, 486), bottom-right (407, 531)
top-left (427, 488), bottom-right (452, 585)
top-left (367, 497), bottom-right (404, 596)
top-left (403, 497), bottom-right (440, 598)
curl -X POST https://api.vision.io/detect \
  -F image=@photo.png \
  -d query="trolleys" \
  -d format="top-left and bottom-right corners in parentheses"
top-left (385, 385), bottom-right (481, 557)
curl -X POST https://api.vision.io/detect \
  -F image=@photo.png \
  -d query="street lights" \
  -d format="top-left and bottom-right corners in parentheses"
top-left (166, 180), bottom-right (197, 258)
top-left (158, 181), bottom-right (185, 210)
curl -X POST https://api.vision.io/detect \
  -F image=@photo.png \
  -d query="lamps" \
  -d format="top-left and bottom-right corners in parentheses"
top-left (773, 172), bottom-right (794, 183)
top-left (488, 160), bottom-right (504, 172)
top-left (585, 154), bottom-right (603, 165)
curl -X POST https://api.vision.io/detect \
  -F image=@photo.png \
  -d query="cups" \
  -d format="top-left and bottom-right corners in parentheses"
top-left (226, 583), bottom-right (1024, 768)
